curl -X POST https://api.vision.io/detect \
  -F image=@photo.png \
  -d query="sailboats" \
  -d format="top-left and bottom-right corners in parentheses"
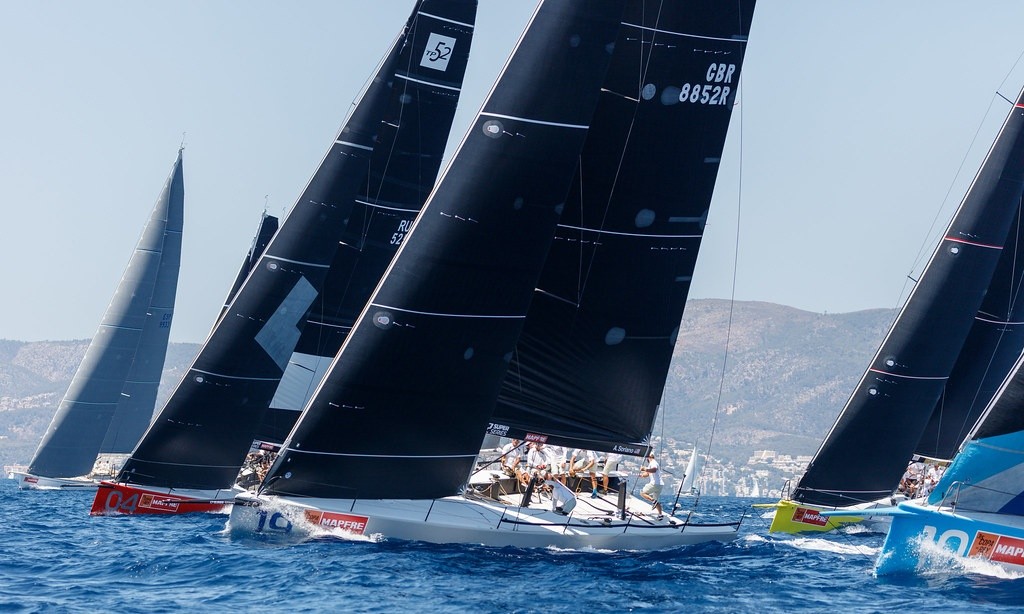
top-left (5, 142), bottom-right (191, 495)
top-left (55, 1), bottom-right (482, 516)
top-left (158, 0), bottom-right (768, 558)
top-left (821, 350), bottom-right (1024, 579)
top-left (198, 209), bottom-right (279, 487)
top-left (749, 51), bottom-right (1024, 534)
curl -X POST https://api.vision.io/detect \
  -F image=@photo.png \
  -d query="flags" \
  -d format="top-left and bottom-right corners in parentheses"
top-left (681, 446), bottom-right (698, 494)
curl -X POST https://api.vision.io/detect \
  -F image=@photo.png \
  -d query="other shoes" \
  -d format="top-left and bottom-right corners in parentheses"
top-left (652, 500), bottom-right (659, 510)
top-left (603, 490), bottom-right (608, 494)
top-left (591, 492), bottom-right (597, 498)
top-left (657, 515), bottom-right (663, 520)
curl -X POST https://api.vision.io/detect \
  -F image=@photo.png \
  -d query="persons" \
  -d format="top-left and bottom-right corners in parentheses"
top-left (641, 452), bottom-right (664, 521)
top-left (500, 439), bottom-right (568, 493)
top-left (601, 452), bottom-right (622, 494)
top-left (97, 460), bottom-right (110, 468)
top-left (570, 449), bottom-right (599, 499)
top-left (899, 461), bottom-right (943, 497)
top-left (249, 450), bottom-right (276, 481)
